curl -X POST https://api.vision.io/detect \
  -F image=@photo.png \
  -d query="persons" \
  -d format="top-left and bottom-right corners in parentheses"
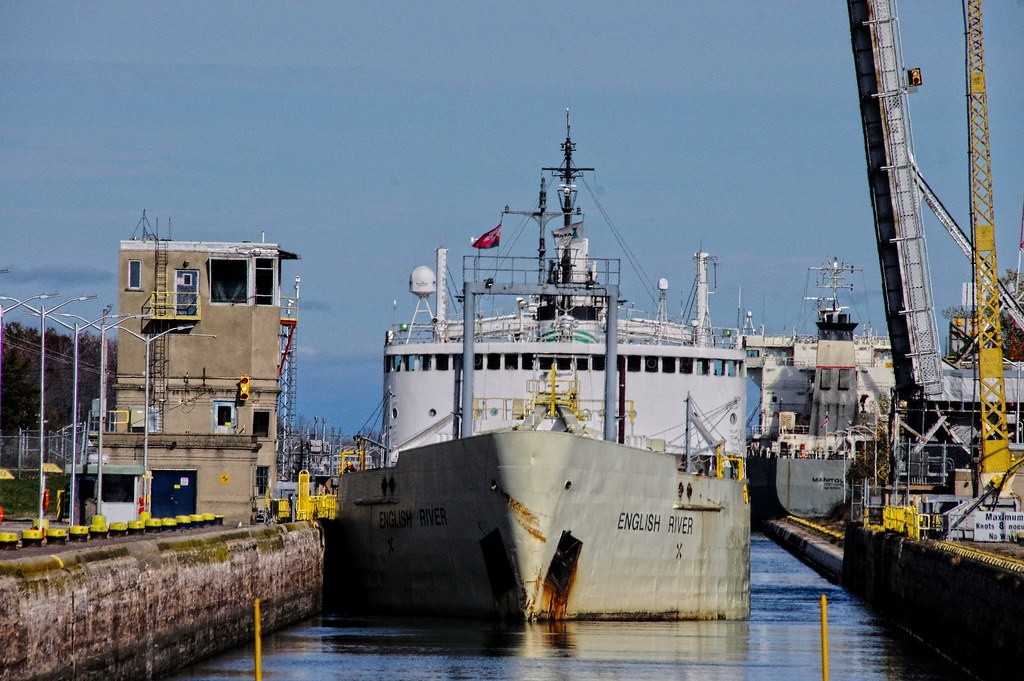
top-left (343, 461), bottom-right (357, 474)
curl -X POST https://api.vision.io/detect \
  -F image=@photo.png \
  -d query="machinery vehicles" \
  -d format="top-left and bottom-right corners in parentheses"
top-left (846, 0), bottom-right (1024, 545)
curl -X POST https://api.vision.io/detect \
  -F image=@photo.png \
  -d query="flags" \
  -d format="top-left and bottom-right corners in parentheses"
top-left (471, 223), bottom-right (502, 249)
top-left (820, 415), bottom-right (830, 429)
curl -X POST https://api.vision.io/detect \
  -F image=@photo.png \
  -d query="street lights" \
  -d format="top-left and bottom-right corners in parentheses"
top-left (59, 313), bottom-right (153, 535)
top-left (109, 325), bottom-right (195, 532)
top-left (22, 312), bottom-right (130, 545)
top-left (0, 295), bottom-right (100, 541)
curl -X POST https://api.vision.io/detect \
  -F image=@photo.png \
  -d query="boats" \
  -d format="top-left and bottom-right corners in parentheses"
top-left (324, 106), bottom-right (746, 622)
top-left (740, 258), bottom-right (1023, 519)
